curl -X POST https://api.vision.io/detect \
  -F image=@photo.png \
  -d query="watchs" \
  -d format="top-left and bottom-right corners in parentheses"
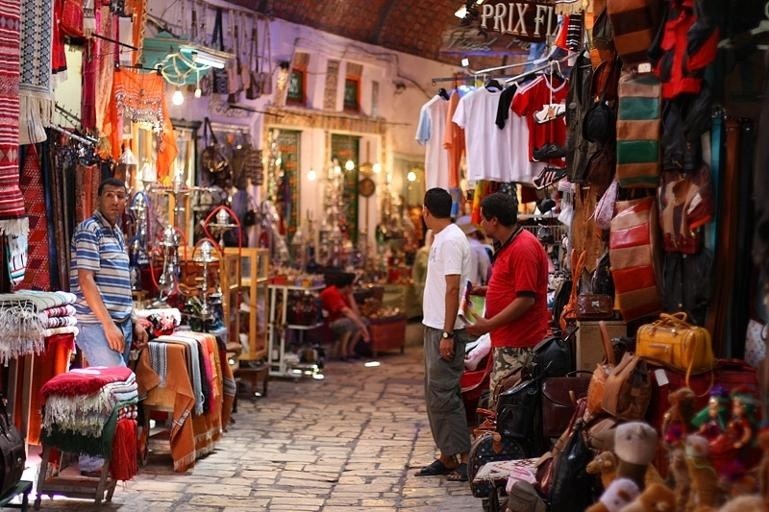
top-left (441, 331), bottom-right (455, 339)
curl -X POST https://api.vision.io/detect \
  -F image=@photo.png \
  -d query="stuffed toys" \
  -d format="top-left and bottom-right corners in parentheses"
top-left (578, 421), bottom-right (768, 512)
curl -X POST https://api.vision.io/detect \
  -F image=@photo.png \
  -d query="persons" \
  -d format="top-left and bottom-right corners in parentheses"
top-left (464, 192), bottom-right (550, 456)
top-left (414, 187), bottom-right (472, 482)
top-left (456, 215), bottom-right (491, 287)
top-left (414, 229), bottom-right (433, 315)
top-left (693, 388), bottom-right (731, 436)
top-left (473, 230), bottom-right (493, 262)
top-left (710, 393), bottom-right (762, 454)
top-left (69, 177), bottom-right (150, 483)
top-left (309, 272), bottom-right (372, 362)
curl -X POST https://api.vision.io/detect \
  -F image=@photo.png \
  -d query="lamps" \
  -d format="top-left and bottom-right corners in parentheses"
top-left (345, 159), bottom-right (355, 171)
top-left (307, 168), bottom-right (317, 182)
top-left (173, 86), bottom-right (184, 105)
top-left (372, 162), bottom-right (382, 173)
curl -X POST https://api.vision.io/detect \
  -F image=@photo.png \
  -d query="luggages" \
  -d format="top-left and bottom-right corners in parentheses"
top-left (654, 353), bottom-right (764, 482)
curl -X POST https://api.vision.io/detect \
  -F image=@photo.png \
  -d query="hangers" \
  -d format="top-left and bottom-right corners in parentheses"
top-left (513, 69), bottom-right (547, 85)
top-left (543, 61), bottom-right (568, 84)
top-left (50, 120), bottom-right (120, 170)
top-left (113, 50), bottom-right (166, 76)
top-left (431, 76), bottom-right (451, 102)
top-left (452, 74), bottom-right (459, 93)
top-left (481, 69), bottom-right (504, 93)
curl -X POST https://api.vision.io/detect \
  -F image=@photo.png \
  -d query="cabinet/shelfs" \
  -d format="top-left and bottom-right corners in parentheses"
top-left (0, 245), bottom-right (269, 512)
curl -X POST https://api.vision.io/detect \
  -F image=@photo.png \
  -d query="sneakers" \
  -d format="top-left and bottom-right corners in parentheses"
top-left (79, 469), bottom-right (111, 479)
top-left (532, 101), bottom-right (567, 126)
top-left (531, 166), bottom-right (567, 191)
top-left (531, 141), bottom-right (566, 163)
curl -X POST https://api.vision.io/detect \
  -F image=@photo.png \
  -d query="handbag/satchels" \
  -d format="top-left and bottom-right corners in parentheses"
top-left (581, 102), bottom-right (617, 143)
top-left (593, 180), bottom-right (620, 231)
top-left (0, 409), bottom-right (28, 501)
top-left (551, 278), bottom-right (573, 329)
top-left (185, 54), bottom-right (275, 106)
top-left (202, 143), bottom-right (292, 261)
top-left (569, 57), bottom-right (593, 101)
top-left (569, 145), bottom-right (594, 183)
top-left (589, 249), bottom-right (615, 295)
top-left (591, 57), bottom-right (622, 104)
top-left (663, 231), bottom-right (699, 254)
top-left (590, 5), bottom-right (616, 52)
top-left (584, 150), bottom-right (615, 186)
top-left (635, 309), bottom-right (715, 377)
top-left (463, 332), bottom-right (651, 512)
top-left (276, 292), bottom-right (326, 364)
top-left (743, 315), bottom-right (769, 372)
top-left (574, 293), bottom-right (615, 322)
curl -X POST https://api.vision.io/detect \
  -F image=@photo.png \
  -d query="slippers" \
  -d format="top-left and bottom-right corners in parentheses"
top-left (446, 460), bottom-right (470, 483)
top-left (413, 456), bottom-right (459, 477)
